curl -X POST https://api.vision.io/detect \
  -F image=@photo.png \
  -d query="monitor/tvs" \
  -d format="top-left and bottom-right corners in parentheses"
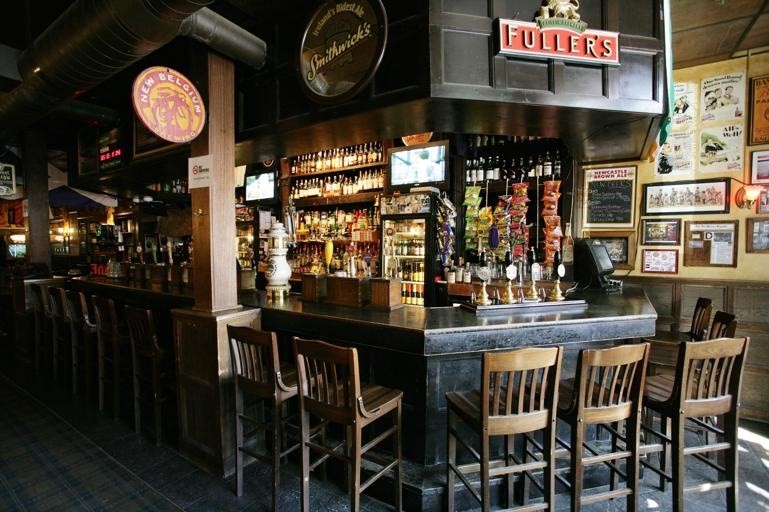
top-left (388, 140), bottom-right (450, 193)
top-left (243, 168), bottom-right (279, 205)
top-left (572, 236), bottom-right (614, 290)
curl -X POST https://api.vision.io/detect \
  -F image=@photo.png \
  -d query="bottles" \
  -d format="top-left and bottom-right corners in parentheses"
top-left (288, 143), bottom-right (383, 175)
top-left (384, 220), bottom-right (424, 305)
top-left (287, 210), bottom-right (380, 276)
top-left (465, 133), bottom-right (561, 185)
top-left (447, 256), bottom-right (473, 285)
top-left (291, 166), bottom-right (385, 201)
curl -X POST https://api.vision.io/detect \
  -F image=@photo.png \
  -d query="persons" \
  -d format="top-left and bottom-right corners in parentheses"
top-left (657, 142), bottom-right (671, 174)
top-left (704, 86), bottom-right (739, 112)
top-left (674, 95), bottom-right (689, 115)
top-left (649, 186), bottom-right (722, 206)
top-left (705, 138), bottom-right (723, 153)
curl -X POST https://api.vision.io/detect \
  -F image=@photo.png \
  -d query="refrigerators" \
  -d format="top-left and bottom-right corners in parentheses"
top-left (378, 192), bottom-right (443, 306)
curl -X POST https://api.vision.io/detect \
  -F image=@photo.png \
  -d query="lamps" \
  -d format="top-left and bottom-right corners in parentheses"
top-left (737, 184), bottom-right (765, 210)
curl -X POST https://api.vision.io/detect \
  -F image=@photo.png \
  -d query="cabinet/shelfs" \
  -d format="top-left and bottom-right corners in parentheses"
top-left (284, 135), bottom-right (389, 280)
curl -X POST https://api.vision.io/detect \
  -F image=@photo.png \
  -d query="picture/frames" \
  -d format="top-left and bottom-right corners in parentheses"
top-left (583, 231), bottom-right (636, 271)
top-left (642, 176), bottom-right (732, 216)
top-left (640, 218), bottom-right (681, 246)
top-left (747, 74), bottom-right (769, 146)
top-left (745, 214), bottom-right (769, 255)
top-left (641, 249), bottom-right (679, 274)
top-left (750, 149), bottom-right (768, 184)
top-left (685, 220), bottom-right (740, 269)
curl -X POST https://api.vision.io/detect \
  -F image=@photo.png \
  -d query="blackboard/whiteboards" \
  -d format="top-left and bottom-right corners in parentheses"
top-left (0, 164), bottom-right (16, 197)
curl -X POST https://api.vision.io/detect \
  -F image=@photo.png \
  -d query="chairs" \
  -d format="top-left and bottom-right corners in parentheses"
top-left (519, 342), bottom-right (650, 512)
top-left (445, 345), bottom-right (564, 512)
top-left (641, 297), bottom-right (738, 466)
top-left (30, 281), bottom-right (179, 449)
top-left (226, 323), bottom-right (328, 512)
top-left (291, 335), bottom-right (407, 512)
top-left (610, 336), bottom-right (752, 512)
